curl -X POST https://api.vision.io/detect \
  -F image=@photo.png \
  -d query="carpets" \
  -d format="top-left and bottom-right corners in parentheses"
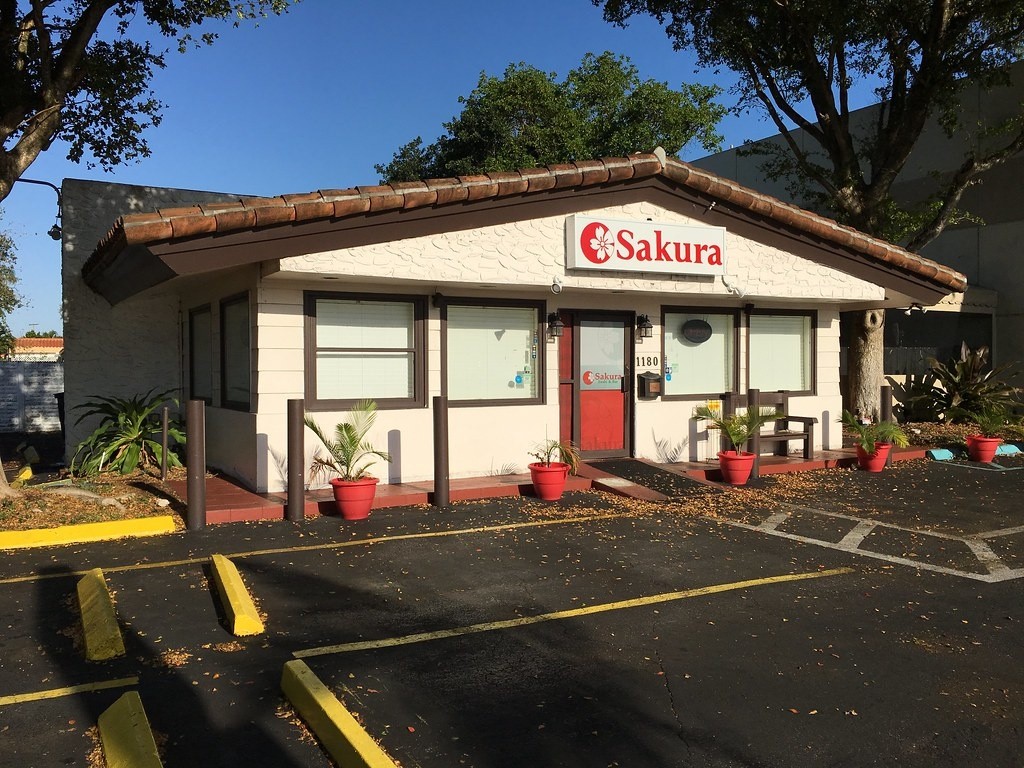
top-left (585, 460), bottom-right (724, 496)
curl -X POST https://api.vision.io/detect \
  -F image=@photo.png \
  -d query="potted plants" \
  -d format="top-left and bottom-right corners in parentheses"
top-left (526, 439), bottom-right (579, 501)
top-left (945, 404), bottom-right (1024, 463)
top-left (304, 398), bottom-right (394, 519)
top-left (694, 405), bottom-right (787, 485)
top-left (837, 409), bottom-right (911, 473)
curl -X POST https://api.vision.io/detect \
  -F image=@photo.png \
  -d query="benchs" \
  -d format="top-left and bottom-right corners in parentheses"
top-left (723, 390), bottom-right (818, 459)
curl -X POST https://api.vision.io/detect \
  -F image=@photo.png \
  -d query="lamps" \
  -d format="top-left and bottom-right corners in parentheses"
top-left (916, 305), bottom-right (928, 314)
top-left (637, 314), bottom-right (653, 338)
top-left (548, 312), bottom-right (564, 337)
top-left (905, 303), bottom-right (914, 316)
